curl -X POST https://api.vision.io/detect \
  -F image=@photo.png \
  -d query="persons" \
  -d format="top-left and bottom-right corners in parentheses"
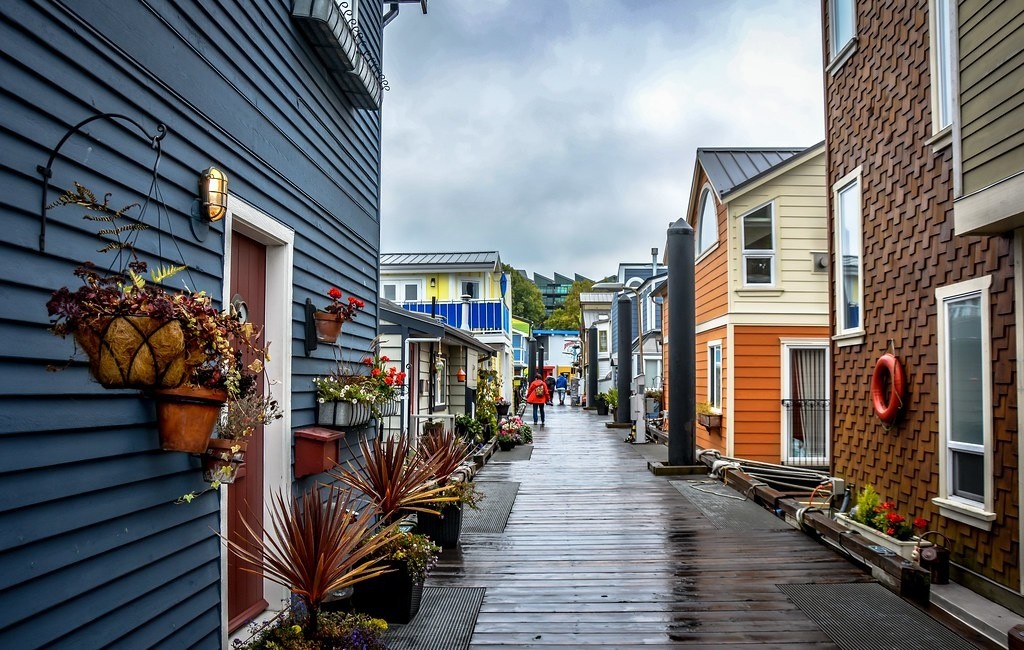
top-left (556, 372), bottom-right (567, 405)
top-left (544, 372), bottom-right (555, 405)
top-left (526, 374), bottom-right (550, 426)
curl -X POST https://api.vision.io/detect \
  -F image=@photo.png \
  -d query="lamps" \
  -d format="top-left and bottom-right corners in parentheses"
top-left (456, 345), bottom-right (467, 382)
top-left (199, 166), bottom-right (228, 223)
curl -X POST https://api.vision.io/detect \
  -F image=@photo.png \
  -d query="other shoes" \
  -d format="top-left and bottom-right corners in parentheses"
top-left (534, 423), bottom-right (537, 425)
top-left (542, 423), bottom-right (545, 425)
top-left (547, 403), bottom-right (550, 405)
top-left (560, 400), bottom-right (563, 405)
top-left (551, 403), bottom-right (553, 406)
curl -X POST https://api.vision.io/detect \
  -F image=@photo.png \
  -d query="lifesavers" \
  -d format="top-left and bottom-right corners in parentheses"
top-left (870, 354), bottom-right (905, 424)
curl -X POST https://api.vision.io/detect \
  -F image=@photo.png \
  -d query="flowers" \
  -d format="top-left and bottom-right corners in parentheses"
top-left (208, 480), bottom-right (409, 650)
top-left (324, 287), bottom-right (365, 322)
top-left (494, 395), bottom-right (533, 445)
top-left (848, 481), bottom-right (928, 541)
top-left (315, 429), bottom-right (463, 587)
top-left (311, 333), bottom-right (410, 404)
top-left (189, 362), bottom-right (258, 401)
top-left (402, 426), bottom-right (488, 520)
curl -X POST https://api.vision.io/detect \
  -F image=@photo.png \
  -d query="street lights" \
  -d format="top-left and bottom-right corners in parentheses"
top-left (592, 282), bottom-right (643, 375)
top-left (564, 337), bottom-right (586, 395)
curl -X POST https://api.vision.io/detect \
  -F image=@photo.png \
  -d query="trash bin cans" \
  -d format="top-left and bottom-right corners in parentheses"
top-left (597, 392), bottom-right (609, 415)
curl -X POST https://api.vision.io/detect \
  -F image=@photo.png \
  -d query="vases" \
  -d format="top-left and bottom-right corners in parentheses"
top-left (834, 512), bottom-right (932, 563)
top-left (315, 312), bottom-right (346, 344)
top-left (495, 405), bottom-right (510, 416)
top-left (499, 441), bottom-right (511, 451)
top-left (376, 399), bottom-right (398, 416)
top-left (154, 386), bottom-right (228, 456)
top-left (334, 53), bottom-right (383, 110)
top-left (510, 440), bottom-right (517, 449)
top-left (292, 0), bottom-right (361, 71)
top-left (351, 558), bottom-right (425, 625)
top-left (393, 501), bottom-right (462, 549)
top-left (318, 401), bottom-right (371, 426)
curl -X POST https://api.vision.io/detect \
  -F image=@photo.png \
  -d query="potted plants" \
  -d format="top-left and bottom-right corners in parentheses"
top-left (697, 400), bottom-right (721, 428)
top-left (478, 368), bottom-right (490, 383)
top-left (173, 394), bottom-right (279, 506)
top-left (594, 392), bottom-right (609, 416)
top-left (602, 387), bottom-right (618, 422)
top-left (490, 369), bottom-right (507, 388)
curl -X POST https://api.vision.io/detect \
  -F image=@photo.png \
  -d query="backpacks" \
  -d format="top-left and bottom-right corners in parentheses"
top-left (536, 383), bottom-right (544, 397)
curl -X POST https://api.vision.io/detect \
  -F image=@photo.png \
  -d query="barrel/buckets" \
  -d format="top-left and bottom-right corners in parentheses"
top-left (918, 531), bottom-right (951, 585)
